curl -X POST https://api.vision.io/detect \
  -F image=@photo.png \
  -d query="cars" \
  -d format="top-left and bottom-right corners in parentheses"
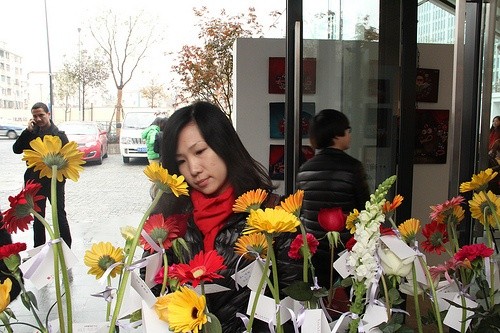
top-left (0, 122), bottom-right (25, 139)
top-left (57, 121), bottom-right (109, 164)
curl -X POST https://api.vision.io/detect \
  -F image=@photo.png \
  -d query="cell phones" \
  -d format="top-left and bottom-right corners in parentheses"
top-left (32, 122), bottom-right (35, 127)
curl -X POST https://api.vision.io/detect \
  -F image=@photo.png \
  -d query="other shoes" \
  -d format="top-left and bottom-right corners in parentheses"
top-left (22, 257), bottom-right (31, 262)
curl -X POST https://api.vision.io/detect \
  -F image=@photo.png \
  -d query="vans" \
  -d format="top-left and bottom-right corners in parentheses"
top-left (116, 109), bottom-right (162, 163)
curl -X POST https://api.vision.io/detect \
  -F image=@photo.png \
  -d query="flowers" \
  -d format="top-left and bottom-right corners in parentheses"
top-left (0, 135), bottom-right (500, 333)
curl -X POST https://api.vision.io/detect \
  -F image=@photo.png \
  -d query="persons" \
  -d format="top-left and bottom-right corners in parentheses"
top-left (138, 101), bottom-right (312, 333)
top-left (13, 102), bottom-right (72, 248)
top-left (142, 116), bottom-right (169, 202)
top-left (295, 108), bottom-right (370, 290)
top-left (489, 116), bottom-right (500, 150)
